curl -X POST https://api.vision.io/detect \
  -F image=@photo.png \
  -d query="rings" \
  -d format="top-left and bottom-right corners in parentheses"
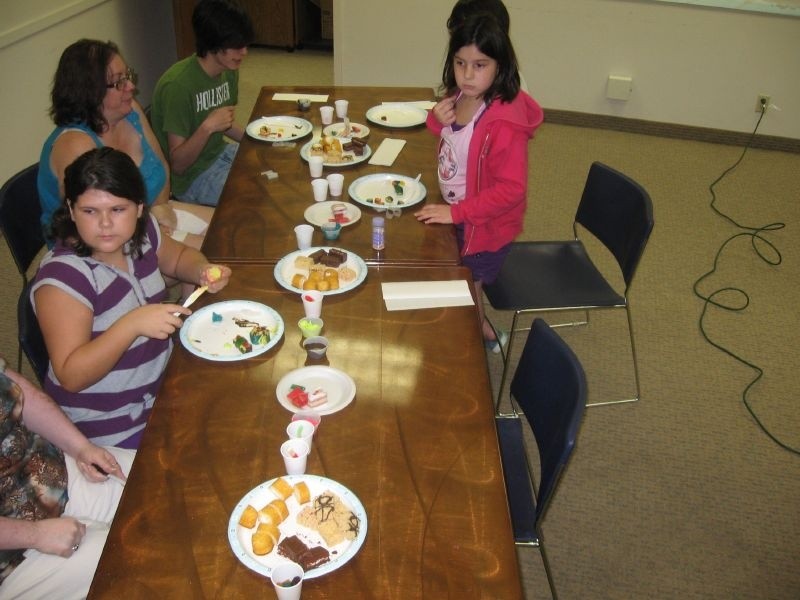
top-left (111, 461), bottom-right (119, 466)
top-left (72, 545), bottom-right (79, 550)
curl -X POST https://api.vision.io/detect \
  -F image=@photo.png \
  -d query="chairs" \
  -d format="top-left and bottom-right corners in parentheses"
top-left (482, 160), bottom-right (653, 408)
top-left (0, 162), bottom-right (52, 390)
top-left (495, 319), bottom-right (588, 600)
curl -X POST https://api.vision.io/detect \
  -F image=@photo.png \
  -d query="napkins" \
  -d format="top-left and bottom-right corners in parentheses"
top-left (272, 93), bottom-right (329, 102)
top-left (383, 101), bottom-right (437, 109)
top-left (368, 138), bottom-right (407, 166)
top-left (381, 280), bottom-right (475, 310)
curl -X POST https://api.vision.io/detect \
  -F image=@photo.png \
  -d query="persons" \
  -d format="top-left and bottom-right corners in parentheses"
top-left (37, 38), bottom-right (216, 303)
top-left (0, 358), bottom-right (136, 600)
top-left (150, 1), bottom-right (255, 208)
top-left (414, 0), bottom-right (544, 353)
top-left (30, 146), bottom-right (232, 450)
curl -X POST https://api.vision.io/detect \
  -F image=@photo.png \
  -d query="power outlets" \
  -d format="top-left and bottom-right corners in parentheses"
top-left (756, 95), bottom-right (770, 113)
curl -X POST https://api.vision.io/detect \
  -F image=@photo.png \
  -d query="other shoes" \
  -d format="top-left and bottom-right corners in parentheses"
top-left (487, 329), bottom-right (509, 353)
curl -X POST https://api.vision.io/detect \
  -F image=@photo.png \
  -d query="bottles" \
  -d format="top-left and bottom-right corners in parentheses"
top-left (372, 217), bottom-right (385, 250)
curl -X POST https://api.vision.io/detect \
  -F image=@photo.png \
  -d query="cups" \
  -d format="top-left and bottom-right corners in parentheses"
top-left (296, 97), bottom-right (312, 111)
top-left (335, 100), bottom-right (349, 119)
top-left (270, 562), bottom-right (304, 600)
top-left (320, 105), bottom-right (334, 123)
top-left (294, 224), bottom-right (314, 249)
top-left (298, 290), bottom-right (328, 360)
top-left (321, 221), bottom-right (342, 240)
top-left (280, 409), bottom-right (321, 475)
top-left (308, 155), bottom-right (345, 201)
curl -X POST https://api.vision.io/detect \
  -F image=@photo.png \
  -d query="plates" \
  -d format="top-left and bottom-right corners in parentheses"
top-left (303, 200), bottom-right (361, 228)
top-left (300, 136), bottom-right (372, 166)
top-left (347, 172), bottom-right (427, 208)
top-left (179, 300), bottom-right (284, 362)
top-left (228, 474), bottom-right (367, 580)
top-left (322, 122), bottom-right (370, 138)
top-left (365, 103), bottom-right (429, 128)
top-left (274, 246), bottom-right (368, 295)
top-left (276, 365), bottom-right (358, 415)
top-left (245, 115), bottom-right (313, 142)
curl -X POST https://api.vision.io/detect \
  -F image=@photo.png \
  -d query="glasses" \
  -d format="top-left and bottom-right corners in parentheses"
top-left (106, 74), bottom-right (135, 91)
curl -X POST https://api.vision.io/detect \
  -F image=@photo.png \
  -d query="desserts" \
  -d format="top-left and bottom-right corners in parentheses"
top-left (276, 536), bottom-right (331, 571)
top-left (234, 326), bottom-right (271, 353)
top-left (341, 136), bottom-right (367, 156)
top-left (287, 384), bottom-right (327, 407)
top-left (308, 248), bottom-right (347, 267)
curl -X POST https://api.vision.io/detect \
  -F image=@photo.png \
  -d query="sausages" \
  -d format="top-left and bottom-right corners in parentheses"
top-left (292, 269), bottom-right (339, 291)
top-left (321, 135), bottom-right (340, 152)
top-left (239, 478), bottom-right (311, 554)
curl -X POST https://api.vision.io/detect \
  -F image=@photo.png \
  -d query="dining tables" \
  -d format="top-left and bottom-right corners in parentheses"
top-left (87, 86), bottom-right (525, 600)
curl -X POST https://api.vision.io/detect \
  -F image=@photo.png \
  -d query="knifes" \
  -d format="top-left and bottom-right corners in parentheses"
top-left (172, 285), bottom-right (208, 316)
